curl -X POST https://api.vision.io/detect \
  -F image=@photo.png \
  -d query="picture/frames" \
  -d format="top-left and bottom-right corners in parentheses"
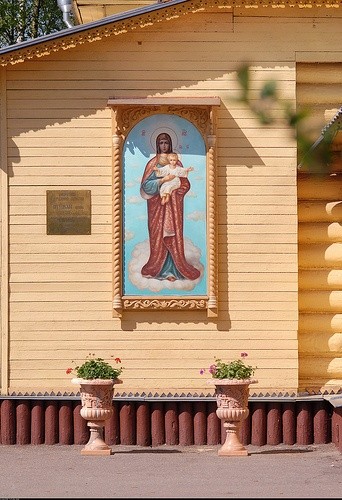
top-left (110, 104), bottom-right (218, 318)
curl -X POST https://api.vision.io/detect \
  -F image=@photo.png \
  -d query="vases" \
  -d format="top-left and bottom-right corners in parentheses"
top-left (72, 379), bottom-right (122, 455)
top-left (213, 378), bottom-right (258, 456)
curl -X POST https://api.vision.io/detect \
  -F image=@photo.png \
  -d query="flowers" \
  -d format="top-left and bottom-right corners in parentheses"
top-left (64, 351), bottom-right (126, 379)
top-left (198, 351), bottom-right (260, 381)
